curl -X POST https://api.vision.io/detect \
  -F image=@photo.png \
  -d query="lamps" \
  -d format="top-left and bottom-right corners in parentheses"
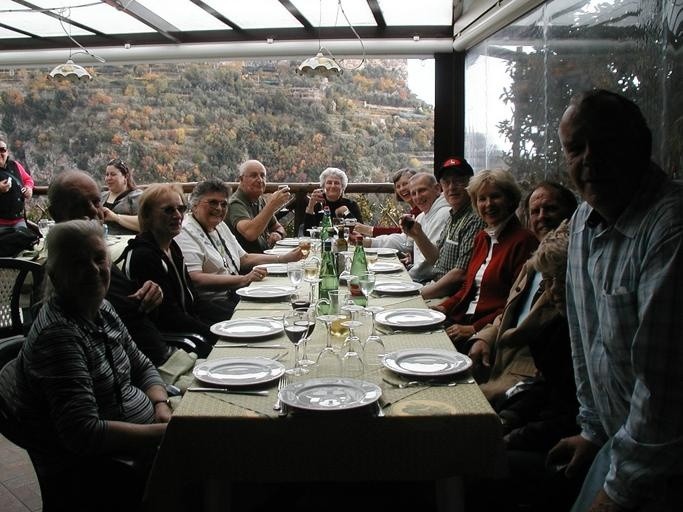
top-left (46, 8), bottom-right (106, 83)
top-left (293, 0), bottom-right (365, 77)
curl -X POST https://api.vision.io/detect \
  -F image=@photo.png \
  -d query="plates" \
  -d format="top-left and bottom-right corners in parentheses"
top-left (190, 238), bottom-right (311, 387)
top-left (278, 377), bottom-right (381, 411)
top-left (363, 246), bottom-right (474, 378)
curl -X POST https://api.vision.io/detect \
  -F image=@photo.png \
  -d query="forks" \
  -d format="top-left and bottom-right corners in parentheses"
top-left (273, 377), bottom-right (290, 410)
top-left (382, 370), bottom-right (476, 388)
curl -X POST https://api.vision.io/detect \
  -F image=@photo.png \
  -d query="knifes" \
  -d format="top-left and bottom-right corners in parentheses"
top-left (187, 385), bottom-right (268, 397)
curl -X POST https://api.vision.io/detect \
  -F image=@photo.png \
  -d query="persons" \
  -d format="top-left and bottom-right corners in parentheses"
top-left (0, 140), bottom-right (292, 511)
top-left (354, 90), bottom-right (683, 512)
top-left (302, 167), bottom-right (364, 239)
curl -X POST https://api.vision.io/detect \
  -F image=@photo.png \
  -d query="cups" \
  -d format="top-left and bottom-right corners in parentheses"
top-left (38, 218), bottom-right (55, 238)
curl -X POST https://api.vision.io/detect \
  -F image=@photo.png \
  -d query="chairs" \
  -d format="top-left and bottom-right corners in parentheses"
top-left (0, 335), bottom-right (146, 512)
top-left (0, 256), bottom-right (46, 370)
top-left (29, 298), bottom-right (207, 362)
top-left (122, 237), bottom-right (236, 352)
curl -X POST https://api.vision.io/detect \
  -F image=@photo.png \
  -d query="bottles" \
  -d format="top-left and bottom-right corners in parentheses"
top-left (317, 206), bottom-right (369, 316)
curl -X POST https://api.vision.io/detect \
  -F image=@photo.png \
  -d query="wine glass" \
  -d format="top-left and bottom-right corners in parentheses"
top-left (305, 225), bottom-right (323, 254)
top-left (277, 185), bottom-right (289, 212)
top-left (399, 214), bottom-right (415, 249)
top-left (284, 264), bottom-right (383, 378)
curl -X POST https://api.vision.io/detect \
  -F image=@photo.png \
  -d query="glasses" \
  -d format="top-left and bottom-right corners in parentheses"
top-left (0, 147), bottom-right (7, 153)
top-left (206, 200), bottom-right (228, 207)
top-left (162, 205), bottom-right (188, 214)
top-left (108, 157), bottom-right (125, 167)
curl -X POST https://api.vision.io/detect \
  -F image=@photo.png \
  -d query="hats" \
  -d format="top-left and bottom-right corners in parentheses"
top-left (434, 155), bottom-right (474, 178)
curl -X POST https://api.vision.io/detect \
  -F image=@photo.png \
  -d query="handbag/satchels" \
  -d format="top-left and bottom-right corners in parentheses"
top-left (0, 226), bottom-right (40, 258)
